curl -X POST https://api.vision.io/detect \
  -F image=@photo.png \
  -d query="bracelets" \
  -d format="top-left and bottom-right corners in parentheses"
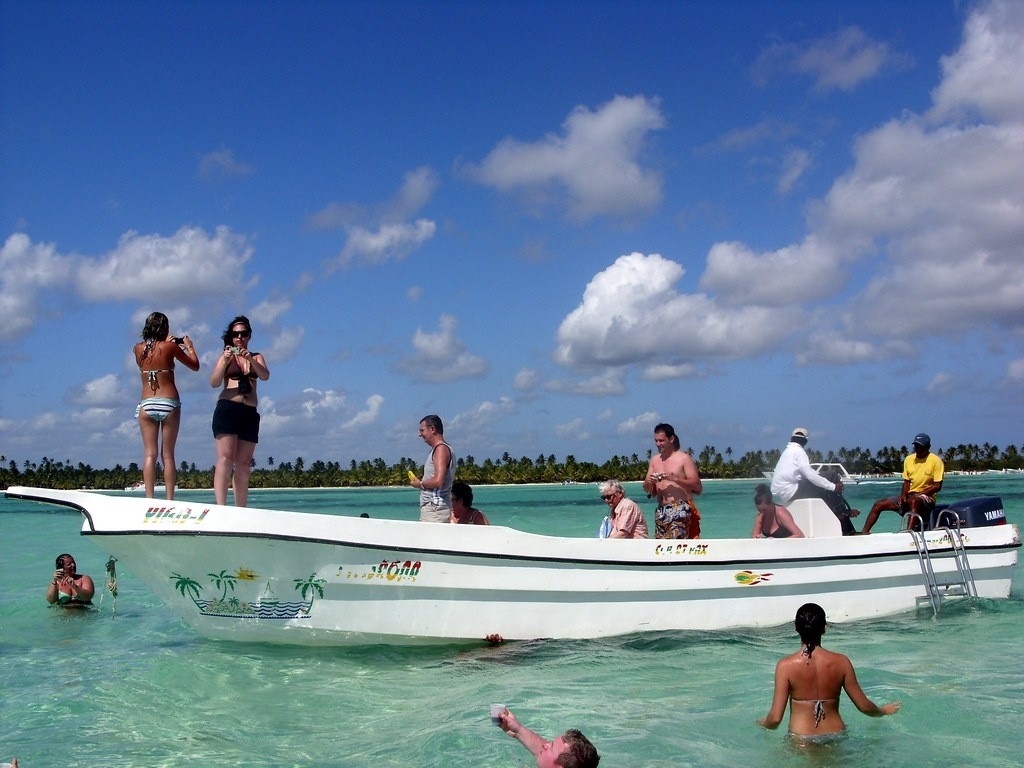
top-left (51, 582), bottom-right (57, 585)
top-left (187, 345), bottom-right (194, 351)
top-left (188, 350), bottom-right (195, 356)
top-left (420, 481), bottom-right (426, 490)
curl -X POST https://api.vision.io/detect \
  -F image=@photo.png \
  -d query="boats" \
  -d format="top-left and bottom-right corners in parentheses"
top-left (4, 481), bottom-right (1020, 653)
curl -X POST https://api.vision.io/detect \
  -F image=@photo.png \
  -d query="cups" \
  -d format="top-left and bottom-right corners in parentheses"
top-left (490, 704), bottom-right (506, 726)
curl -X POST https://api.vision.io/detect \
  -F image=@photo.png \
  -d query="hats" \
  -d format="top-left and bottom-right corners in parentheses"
top-left (792, 427), bottom-right (808, 438)
top-left (911, 433), bottom-right (930, 447)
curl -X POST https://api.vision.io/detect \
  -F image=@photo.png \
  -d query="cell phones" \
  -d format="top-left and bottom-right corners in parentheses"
top-left (407, 471), bottom-right (415, 480)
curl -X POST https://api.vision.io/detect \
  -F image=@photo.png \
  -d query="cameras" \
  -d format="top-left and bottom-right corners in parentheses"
top-left (175, 337), bottom-right (184, 344)
top-left (653, 473), bottom-right (664, 482)
top-left (229, 347), bottom-right (241, 354)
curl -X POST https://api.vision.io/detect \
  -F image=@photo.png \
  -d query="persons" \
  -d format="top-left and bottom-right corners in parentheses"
top-left (642, 422), bottom-right (703, 540)
top-left (210, 315), bottom-right (271, 507)
top-left (860, 433), bottom-right (945, 535)
top-left (488, 708), bottom-right (601, 768)
top-left (410, 415), bottom-right (456, 523)
top-left (450, 482), bottom-right (490, 525)
top-left (598, 480), bottom-right (649, 539)
top-left (132, 312), bottom-right (200, 501)
top-left (46, 554), bottom-right (94, 605)
top-left (770, 427), bottom-right (861, 536)
top-left (757, 604), bottom-right (902, 746)
top-left (883, 473), bottom-right (896, 477)
top-left (752, 483), bottom-right (806, 538)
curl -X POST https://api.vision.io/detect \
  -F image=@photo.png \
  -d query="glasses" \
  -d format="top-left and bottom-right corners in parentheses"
top-left (601, 491), bottom-right (618, 500)
top-left (232, 330), bottom-right (250, 338)
